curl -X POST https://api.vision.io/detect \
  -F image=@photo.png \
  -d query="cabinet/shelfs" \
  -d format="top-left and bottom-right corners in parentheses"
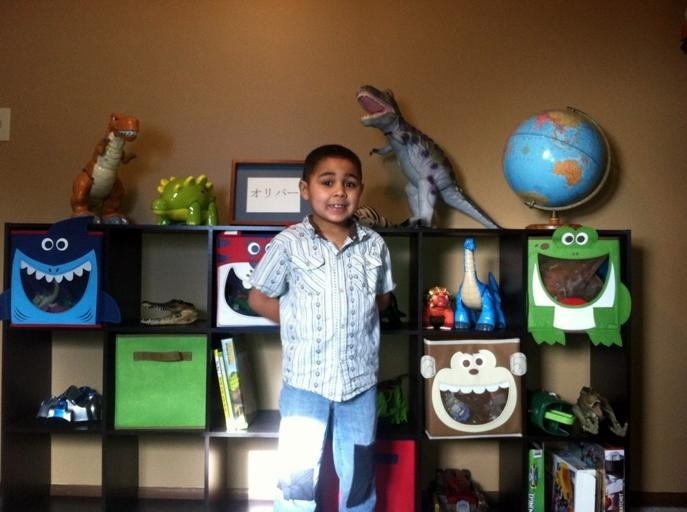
top-left (0, 221), bottom-right (645, 511)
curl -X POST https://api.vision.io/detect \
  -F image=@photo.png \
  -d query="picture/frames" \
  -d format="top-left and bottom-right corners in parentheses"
top-left (231, 161), bottom-right (315, 226)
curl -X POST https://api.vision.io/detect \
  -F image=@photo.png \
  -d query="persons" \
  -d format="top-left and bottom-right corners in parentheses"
top-left (245, 142), bottom-right (397, 512)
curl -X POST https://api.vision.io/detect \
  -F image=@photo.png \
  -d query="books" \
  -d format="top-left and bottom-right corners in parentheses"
top-left (220, 336), bottom-right (258, 431)
top-left (214, 348), bottom-right (231, 432)
top-left (218, 351), bottom-right (238, 431)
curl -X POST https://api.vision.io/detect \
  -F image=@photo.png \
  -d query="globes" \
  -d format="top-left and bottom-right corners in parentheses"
top-left (502, 106), bottom-right (614, 230)
